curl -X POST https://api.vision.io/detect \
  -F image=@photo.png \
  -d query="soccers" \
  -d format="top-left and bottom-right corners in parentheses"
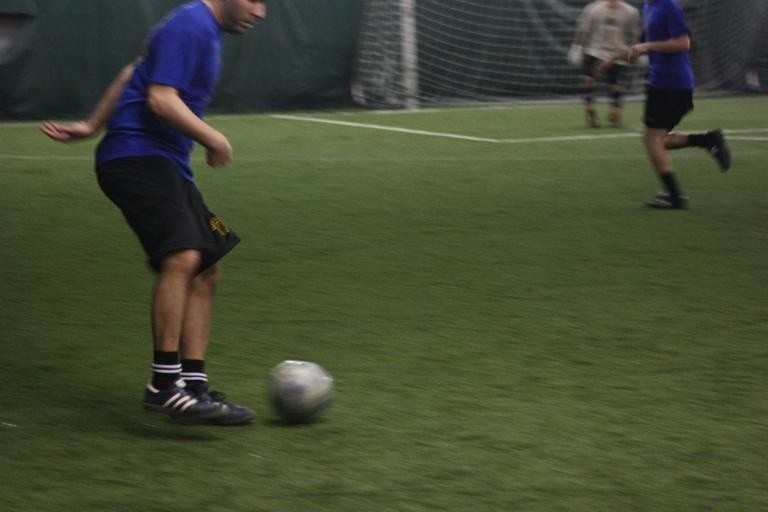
top-left (264, 358), bottom-right (337, 424)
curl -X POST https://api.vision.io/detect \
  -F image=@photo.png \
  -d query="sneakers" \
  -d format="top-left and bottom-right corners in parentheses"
top-left (143, 377), bottom-right (256, 425)
top-left (705, 129), bottom-right (730, 171)
top-left (645, 191), bottom-right (689, 209)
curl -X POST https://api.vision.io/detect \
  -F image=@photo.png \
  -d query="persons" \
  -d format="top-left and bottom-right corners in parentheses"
top-left (596, 0), bottom-right (733, 211)
top-left (39, 1), bottom-right (268, 426)
top-left (565, 1), bottom-right (644, 128)
